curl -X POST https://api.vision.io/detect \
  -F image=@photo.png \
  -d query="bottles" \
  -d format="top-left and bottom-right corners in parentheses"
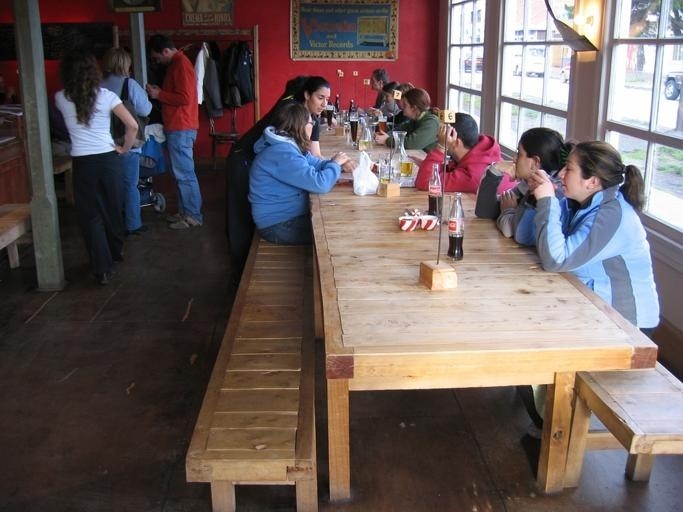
top-left (448, 192), bottom-right (465, 260)
top-left (391, 130), bottom-right (410, 175)
top-left (334, 94), bottom-right (379, 141)
top-left (427, 164), bottom-right (443, 221)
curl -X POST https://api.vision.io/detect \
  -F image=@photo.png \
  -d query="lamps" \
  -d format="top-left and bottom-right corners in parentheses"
top-left (573, 14), bottom-right (592, 27)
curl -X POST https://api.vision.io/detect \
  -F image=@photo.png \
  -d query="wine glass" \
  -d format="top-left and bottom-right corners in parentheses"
top-left (326, 106), bottom-right (388, 148)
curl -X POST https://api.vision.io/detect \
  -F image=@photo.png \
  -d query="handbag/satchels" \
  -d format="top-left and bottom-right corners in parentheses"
top-left (115, 100), bottom-right (147, 148)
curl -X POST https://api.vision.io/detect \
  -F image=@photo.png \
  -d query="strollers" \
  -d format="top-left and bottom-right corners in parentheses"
top-left (140, 129), bottom-right (172, 215)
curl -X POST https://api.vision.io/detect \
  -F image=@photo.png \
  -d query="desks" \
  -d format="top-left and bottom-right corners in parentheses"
top-left (308, 114), bottom-right (658, 503)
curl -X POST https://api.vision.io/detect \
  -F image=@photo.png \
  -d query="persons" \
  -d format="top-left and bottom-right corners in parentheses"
top-left (248, 102), bottom-right (358, 246)
top-left (415, 112), bottom-right (504, 194)
top-left (369, 68), bottom-right (442, 154)
top-left (53, 54), bottom-right (139, 286)
top-left (221, 75), bottom-right (331, 251)
top-left (474, 126), bottom-right (580, 238)
top-left (514, 139), bottom-right (662, 440)
top-left (145, 33), bottom-right (204, 231)
top-left (95, 46), bottom-right (153, 235)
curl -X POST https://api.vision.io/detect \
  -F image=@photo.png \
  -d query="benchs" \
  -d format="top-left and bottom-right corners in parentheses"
top-left (185, 224), bottom-right (319, 512)
top-left (563, 360), bottom-right (683, 489)
top-left (0, 203), bottom-right (32, 269)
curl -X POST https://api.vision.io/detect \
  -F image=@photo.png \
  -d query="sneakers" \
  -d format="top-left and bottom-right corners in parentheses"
top-left (100, 212), bottom-right (203, 285)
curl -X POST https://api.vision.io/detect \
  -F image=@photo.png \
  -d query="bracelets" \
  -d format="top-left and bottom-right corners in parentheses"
top-left (438, 143), bottom-right (448, 151)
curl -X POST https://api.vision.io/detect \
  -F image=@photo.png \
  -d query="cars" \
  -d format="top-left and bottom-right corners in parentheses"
top-left (561, 65), bottom-right (570, 85)
top-left (463, 53), bottom-right (487, 74)
top-left (663, 64), bottom-right (683, 98)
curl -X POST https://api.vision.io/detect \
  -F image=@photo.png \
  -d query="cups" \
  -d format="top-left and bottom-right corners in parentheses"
top-left (524, 175), bottom-right (558, 210)
top-left (401, 162), bottom-right (412, 177)
top-left (379, 153), bottom-right (392, 182)
top-left (398, 212), bottom-right (439, 232)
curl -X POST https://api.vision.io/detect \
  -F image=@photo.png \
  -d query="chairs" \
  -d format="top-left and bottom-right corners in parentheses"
top-left (208, 107), bottom-right (240, 171)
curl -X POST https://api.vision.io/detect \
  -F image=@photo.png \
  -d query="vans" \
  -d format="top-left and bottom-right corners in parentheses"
top-left (511, 45), bottom-right (546, 78)
